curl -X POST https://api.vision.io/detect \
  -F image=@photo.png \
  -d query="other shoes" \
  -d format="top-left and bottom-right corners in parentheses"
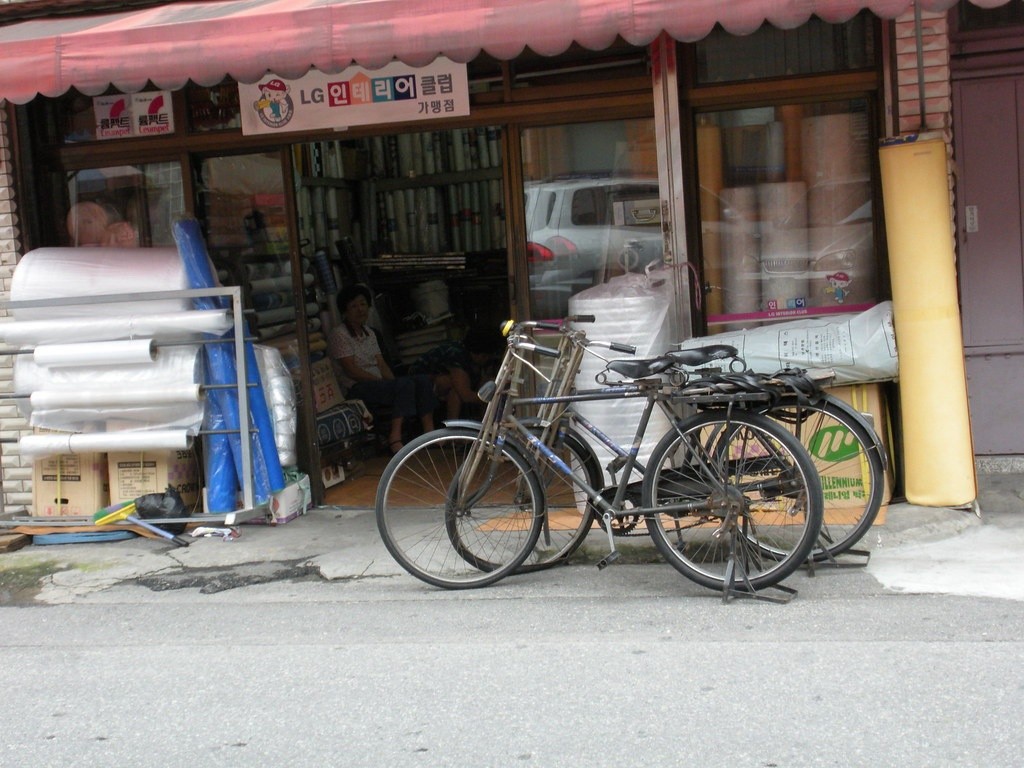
top-left (385, 440), bottom-right (403, 457)
top-left (429, 440), bottom-right (457, 450)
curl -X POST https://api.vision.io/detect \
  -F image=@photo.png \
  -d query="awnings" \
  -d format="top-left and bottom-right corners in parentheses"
top-left (0, 0), bottom-right (1008, 105)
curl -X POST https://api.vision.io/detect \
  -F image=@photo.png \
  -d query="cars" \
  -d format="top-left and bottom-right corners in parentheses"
top-left (736, 171), bottom-right (886, 304)
top-left (521, 173), bottom-right (763, 340)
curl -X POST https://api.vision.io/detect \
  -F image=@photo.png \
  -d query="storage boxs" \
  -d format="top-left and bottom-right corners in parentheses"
top-left (198, 353), bottom-right (382, 526)
top-left (30, 456), bottom-right (110, 525)
top-left (106, 449), bottom-right (202, 513)
top-left (692, 385), bottom-right (897, 510)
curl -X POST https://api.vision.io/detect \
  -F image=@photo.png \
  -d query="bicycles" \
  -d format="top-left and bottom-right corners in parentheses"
top-left (372, 314), bottom-right (887, 605)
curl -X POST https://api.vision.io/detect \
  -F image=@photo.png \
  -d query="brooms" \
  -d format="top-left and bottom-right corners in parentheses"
top-left (93, 500), bottom-right (190, 546)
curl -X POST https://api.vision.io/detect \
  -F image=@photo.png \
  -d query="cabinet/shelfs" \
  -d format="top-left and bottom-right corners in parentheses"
top-left (312, 123), bottom-right (506, 287)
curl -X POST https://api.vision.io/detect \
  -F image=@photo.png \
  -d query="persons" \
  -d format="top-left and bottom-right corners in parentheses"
top-left (326, 286), bottom-right (451, 456)
top-left (407, 328), bottom-right (496, 448)
